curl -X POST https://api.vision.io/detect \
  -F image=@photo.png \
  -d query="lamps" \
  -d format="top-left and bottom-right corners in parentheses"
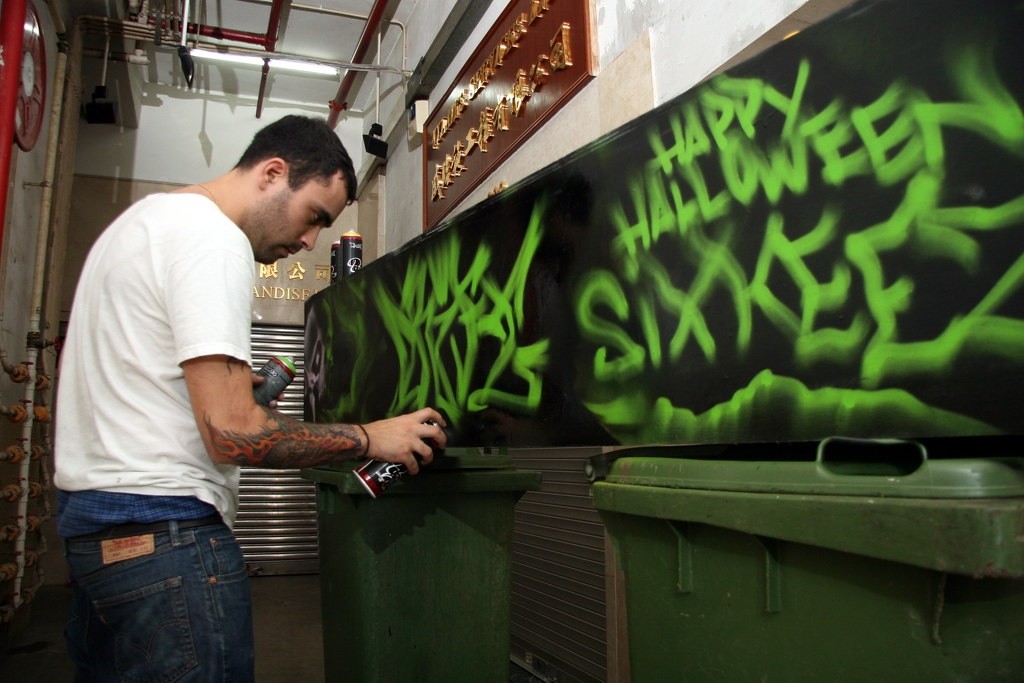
top-left (363, 19), bottom-right (388, 158)
top-left (177, 0), bottom-right (194, 89)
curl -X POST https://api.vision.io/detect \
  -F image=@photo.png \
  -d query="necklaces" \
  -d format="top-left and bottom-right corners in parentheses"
top-left (200, 184), bottom-right (217, 204)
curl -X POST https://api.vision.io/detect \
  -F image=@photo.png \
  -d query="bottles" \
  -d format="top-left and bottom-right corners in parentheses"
top-left (353, 419), bottom-right (441, 498)
top-left (253, 355), bottom-right (296, 409)
top-left (330, 228), bottom-right (364, 286)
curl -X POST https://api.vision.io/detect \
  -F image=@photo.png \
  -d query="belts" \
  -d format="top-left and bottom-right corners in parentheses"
top-left (65, 512), bottom-right (223, 542)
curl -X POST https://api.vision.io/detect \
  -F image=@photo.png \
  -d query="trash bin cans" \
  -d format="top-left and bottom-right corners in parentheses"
top-left (589, 433), bottom-right (1024, 683)
top-left (300, 460), bottom-right (541, 682)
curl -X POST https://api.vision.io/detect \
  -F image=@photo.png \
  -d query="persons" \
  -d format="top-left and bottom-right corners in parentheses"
top-left (54, 115), bottom-right (446, 682)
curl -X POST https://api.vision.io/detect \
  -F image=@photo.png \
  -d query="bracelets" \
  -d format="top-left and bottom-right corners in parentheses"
top-left (357, 424), bottom-right (370, 460)
